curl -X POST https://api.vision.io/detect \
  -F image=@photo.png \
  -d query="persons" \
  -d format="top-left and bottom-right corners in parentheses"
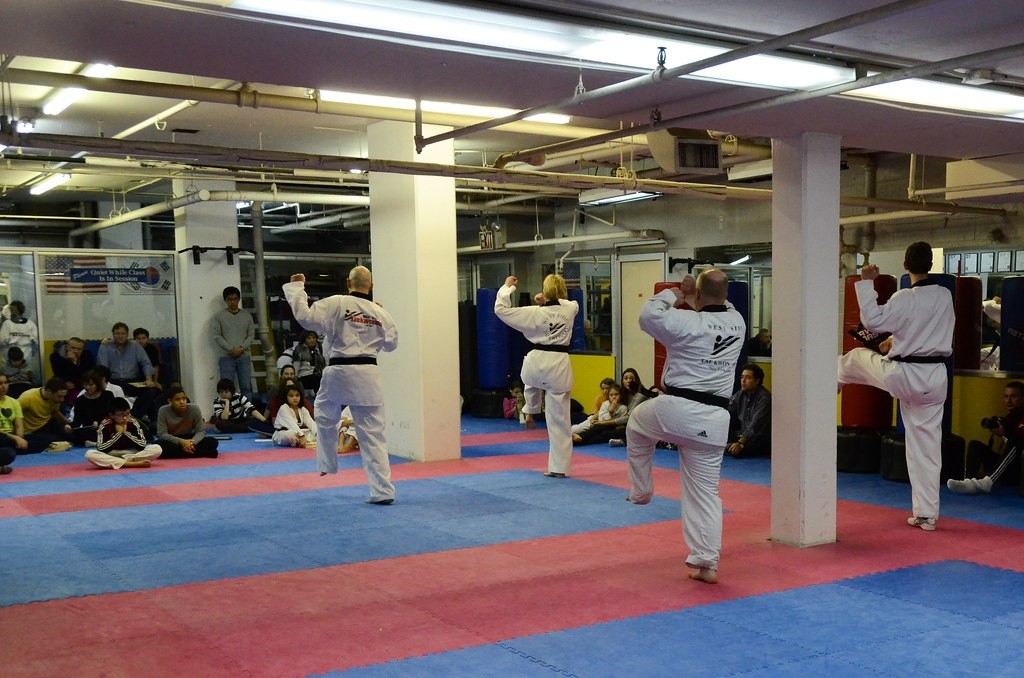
top-left (837, 241), bottom-right (955, 531)
top-left (292, 330), bottom-right (325, 393)
top-left (503, 381), bottom-right (526, 424)
top-left (0, 374), bottom-right (27, 472)
top-left (337, 405), bottom-right (358, 452)
top-left (211, 287), bottom-right (256, 401)
top-left (283, 266), bottom-right (398, 503)
top-left (726, 364), bottom-right (771, 460)
top-left (248, 364), bottom-right (317, 449)
top-left (50, 337), bottom-right (95, 417)
top-left (0, 301), bottom-right (38, 398)
top-left (626, 270), bottom-right (746, 584)
top-left (212, 379), bottom-right (266, 432)
top-left (571, 368), bottom-right (647, 447)
top-left (494, 274), bottom-right (579, 478)
top-left (748, 328), bottom-right (771, 357)
top-left (15, 377), bottom-right (67, 456)
top-left (946, 381), bottom-right (1024, 494)
top-left (64, 321), bottom-right (218, 469)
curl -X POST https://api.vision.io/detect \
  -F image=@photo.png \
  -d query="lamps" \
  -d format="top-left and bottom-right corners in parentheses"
top-left (44, 62), bottom-right (120, 115)
top-left (578, 187), bottom-right (662, 206)
top-left (29, 169), bottom-right (71, 195)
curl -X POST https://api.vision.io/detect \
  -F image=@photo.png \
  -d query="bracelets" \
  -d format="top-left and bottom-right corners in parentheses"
top-left (738, 442), bottom-right (744, 448)
top-left (240, 346), bottom-right (244, 350)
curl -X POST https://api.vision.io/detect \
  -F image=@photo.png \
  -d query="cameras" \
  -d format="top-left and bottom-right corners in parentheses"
top-left (981, 415), bottom-right (1004, 429)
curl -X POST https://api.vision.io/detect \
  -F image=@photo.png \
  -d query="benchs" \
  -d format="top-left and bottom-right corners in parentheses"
top-left (52, 336), bottom-right (183, 388)
